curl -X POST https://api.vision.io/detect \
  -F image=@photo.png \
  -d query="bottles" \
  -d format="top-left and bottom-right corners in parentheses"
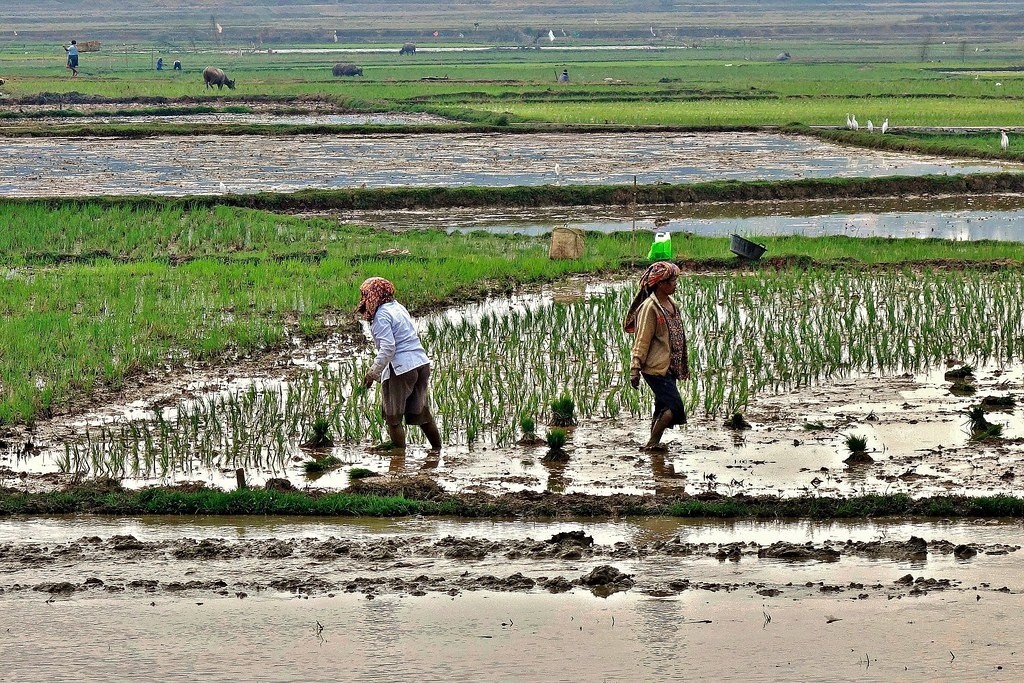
top-left (654, 231), bottom-right (671, 252)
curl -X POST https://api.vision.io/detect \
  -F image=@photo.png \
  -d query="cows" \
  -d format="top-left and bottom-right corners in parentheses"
top-left (331, 62), bottom-right (364, 77)
top-left (775, 51), bottom-right (791, 61)
top-left (399, 43), bottom-right (416, 55)
top-left (203, 67), bottom-right (236, 93)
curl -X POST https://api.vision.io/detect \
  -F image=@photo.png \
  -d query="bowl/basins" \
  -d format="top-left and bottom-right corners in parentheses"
top-left (730, 234), bottom-right (768, 259)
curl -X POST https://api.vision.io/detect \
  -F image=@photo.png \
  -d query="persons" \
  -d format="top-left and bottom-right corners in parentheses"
top-left (563, 70), bottom-right (569, 81)
top-left (173, 59), bottom-right (182, 69)
top-left (356, 277), bottom-right (442, 449)
top-left (626, 262), bottom-right (690, 448)
top-left (156, 57), bottom-right (164, 70)
top-left (63, 40), bottom-right (79, 76)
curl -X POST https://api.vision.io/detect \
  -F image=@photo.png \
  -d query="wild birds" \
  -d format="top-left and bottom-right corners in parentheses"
top-left (998, 130), bottom-right (1009, 150)
top-left (845, 114), bottom-right (859, 131)
top-left (868, 118), bottom-right (889, 133)
